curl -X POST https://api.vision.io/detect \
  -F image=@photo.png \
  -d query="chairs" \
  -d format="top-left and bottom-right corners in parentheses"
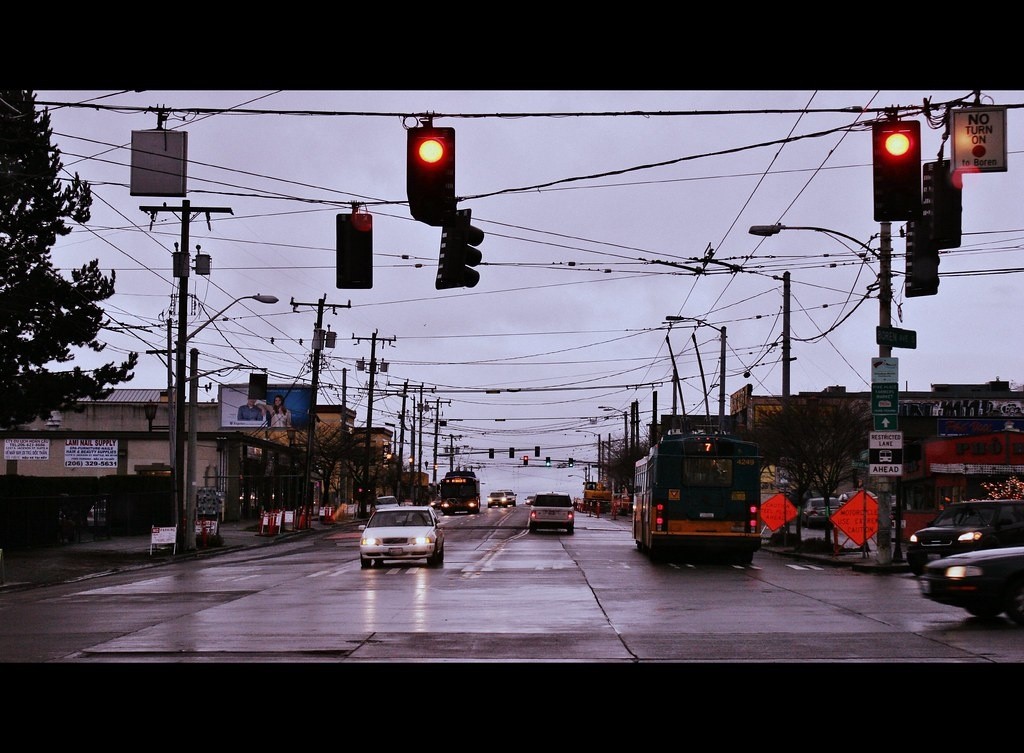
top-left (412, 513), bottom-right (428, 525)
top-left (380, 511), bottom-right (397, 525)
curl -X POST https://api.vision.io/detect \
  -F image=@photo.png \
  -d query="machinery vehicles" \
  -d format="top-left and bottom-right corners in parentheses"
top-left (583, 482), bottom-right (612, 514)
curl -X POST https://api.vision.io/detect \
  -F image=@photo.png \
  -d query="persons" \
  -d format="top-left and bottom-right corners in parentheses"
top-left (237, 397), bottom-right (263, 421)
top-left (256, 395), bottom-right (291, 427)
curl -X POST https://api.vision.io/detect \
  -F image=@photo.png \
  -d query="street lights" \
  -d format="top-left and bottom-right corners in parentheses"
top-left (598, 405), bottom-right (628, 467)
top-left (174, 293), bottom-right (278, 554)
top-left (664, 315), bottom-right (725, 436)
top-left (749, 221), bottom-right (894, 564)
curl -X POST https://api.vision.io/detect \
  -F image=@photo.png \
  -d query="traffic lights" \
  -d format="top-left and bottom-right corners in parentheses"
top-left (872, 119), bottom-right (925, 221)
top-left (546, 457), bottom-right (551, 468)
top-left (435, 209), bottom-right (485, 290)
top-left (569, 458), bottom-right (573, 468)
top-left (407, 127), bottom-right (456, 226)
top-left (523, 456), bottom-right (528, 465)
top-left (905, 161), bottom-right (962, 298)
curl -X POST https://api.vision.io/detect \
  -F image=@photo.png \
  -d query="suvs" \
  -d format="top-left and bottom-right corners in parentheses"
top-left (905, 499), bottom-right (1024, 576)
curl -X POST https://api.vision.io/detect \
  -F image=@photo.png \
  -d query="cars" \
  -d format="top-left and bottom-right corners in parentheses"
top-left (918, 547), bottom-right (1024, 623)
top-left (487, 490), bottom-right (517, 508)
top-left (431, 497), bottom-right (441, 509)
top-left (359, 496), bottom-right (445, 570)
top-left (801, 489), bottom-right (877, 530)
top-left (524, 490), bottom-right (575, 534)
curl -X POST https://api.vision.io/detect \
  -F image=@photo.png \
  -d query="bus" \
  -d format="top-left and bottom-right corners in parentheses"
top-left (441, 445), bottom-right (480, 515)
top-left (630, 333), bottom-right (764, 567)
